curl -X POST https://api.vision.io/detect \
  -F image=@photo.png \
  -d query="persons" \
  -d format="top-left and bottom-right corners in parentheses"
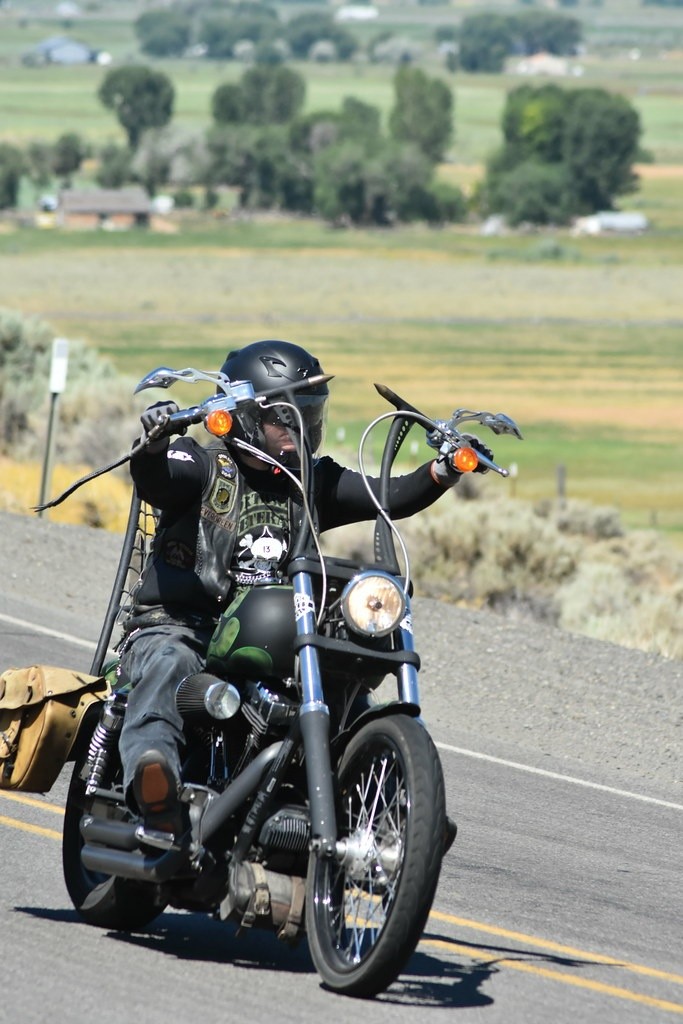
top-left (120, 342), bottom-right (494, 855)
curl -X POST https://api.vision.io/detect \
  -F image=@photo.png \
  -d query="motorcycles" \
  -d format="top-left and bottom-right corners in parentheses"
top-left (0, 369), bottom-right (523, 997)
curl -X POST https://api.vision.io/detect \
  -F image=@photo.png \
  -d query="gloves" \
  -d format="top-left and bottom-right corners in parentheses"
top-left (140, 401), bottom-right (188, 442)
top-left (444, 432), bottom-right (493, 473)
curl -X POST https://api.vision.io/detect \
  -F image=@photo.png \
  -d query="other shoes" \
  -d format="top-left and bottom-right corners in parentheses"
top-left (132, 749), bottom-right (178, 834)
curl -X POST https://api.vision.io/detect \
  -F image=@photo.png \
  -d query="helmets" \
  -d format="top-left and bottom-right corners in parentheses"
top-left (216, 341), bottom-right (329, 471)
